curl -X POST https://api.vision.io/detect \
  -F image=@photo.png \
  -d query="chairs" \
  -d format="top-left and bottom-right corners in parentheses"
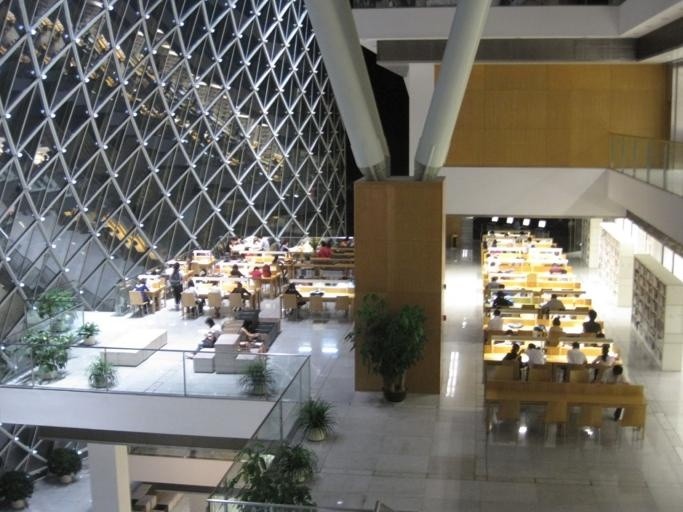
top-left (483, 232), bottom-right (647, 444)
top-left (129, 261), bottom-right (349, 323)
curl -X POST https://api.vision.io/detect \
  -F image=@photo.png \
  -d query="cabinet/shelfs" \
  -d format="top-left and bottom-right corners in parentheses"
top-left (600, 223), bottom-right (635, 307)
top-left (632, 254), bottom-right (683, 373)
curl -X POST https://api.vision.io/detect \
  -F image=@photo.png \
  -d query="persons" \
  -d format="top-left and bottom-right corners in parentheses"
top-left (134, 278), bottom-right (152, 315)
top-left (234, 321), bottom-right (267, 354)
top-left (249, 266), bottom-right (264, 301)
top-left (481, 226), bottom-right (628, 386)
top-left (168, 262), bottom-right (184, 309)
top-left (207, 280), bottom-right (223, 318)
top-left (185, 280), bottom-right (203, 314)
top-left (224, 232), bottom-right (354, 264)
top-left (229, 281), bottom-right (251, 311)
top-left (230, 263), bottom-right (248, 279)
top-left (182, 317), bottom-right (222, 361)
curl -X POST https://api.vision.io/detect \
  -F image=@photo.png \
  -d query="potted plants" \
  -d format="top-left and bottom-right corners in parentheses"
top-left (297, 399), bottom-right (337, 442)
top-left (270, 440), bottom-right (318, 483)
top-left (344, 293), bottom-right (432, 402)
top-left (19, 288), bottom-right (117, 391)
top-left (48, 448), bottom-right (82, 484)
top-left (0, 471), bottom-right (35, 511)
top-left (239, 360), bottom-right (276, 397)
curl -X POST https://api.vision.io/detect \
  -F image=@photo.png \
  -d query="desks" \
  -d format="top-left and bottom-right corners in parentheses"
top-left (287, 247), bottom-right (354, 299)
